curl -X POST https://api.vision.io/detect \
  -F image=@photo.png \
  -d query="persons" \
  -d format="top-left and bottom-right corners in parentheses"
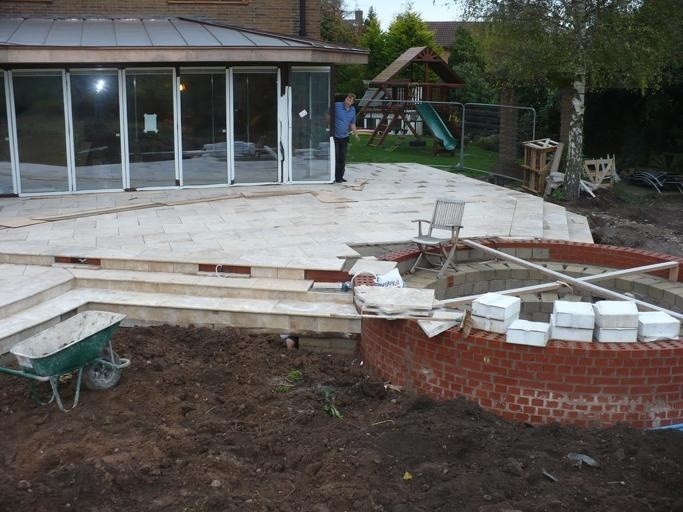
top-left (325, 93), bottom-right (360, 183)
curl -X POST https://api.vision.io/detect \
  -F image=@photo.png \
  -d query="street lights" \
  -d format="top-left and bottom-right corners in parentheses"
top-left (95, 80), bottom-right (105, 122)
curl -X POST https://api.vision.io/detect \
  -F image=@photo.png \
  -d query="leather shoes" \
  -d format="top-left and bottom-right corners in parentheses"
top-left (335, 178), bottom-right (347, 183)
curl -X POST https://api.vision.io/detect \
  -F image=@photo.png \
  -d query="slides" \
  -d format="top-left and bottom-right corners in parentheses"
top-left (414, 103), bottom-right (458, 151)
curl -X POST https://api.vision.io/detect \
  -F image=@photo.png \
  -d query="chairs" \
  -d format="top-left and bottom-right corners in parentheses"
top-left (409, 197), bottom-right (465, 280)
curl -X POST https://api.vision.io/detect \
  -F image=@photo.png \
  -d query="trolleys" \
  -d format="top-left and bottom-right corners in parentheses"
top-left (0, 309), bottom-right (133, 413)
